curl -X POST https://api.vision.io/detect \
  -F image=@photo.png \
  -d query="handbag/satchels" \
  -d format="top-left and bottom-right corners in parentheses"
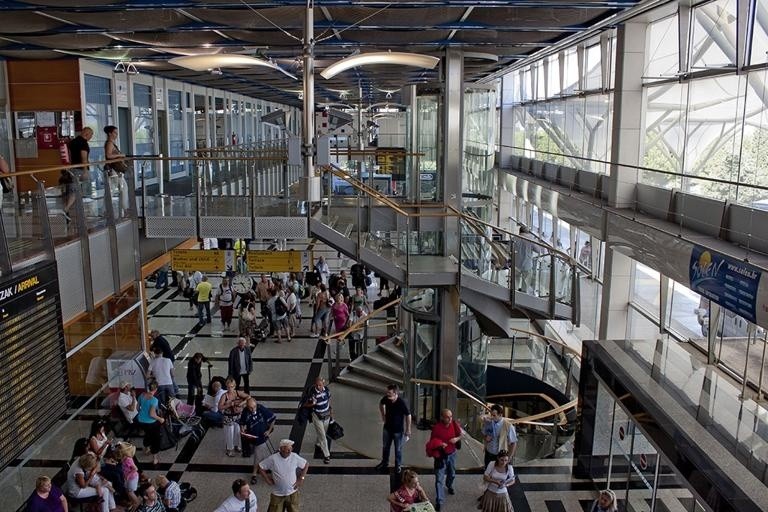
top-left (184, 288), bottom-right (194, 298)
top-left (456, 436), bottom-right (461, 449)
top-left (160, 423), bottom-right (178, 451)
top-left (242, 309), bottom-right (255, 321)
top-left (275, 298), bottom-right (286, 316)
top-left (328, 420), bottom-right (344, 440)
top-left (107, 151), bottom-right (128, 172)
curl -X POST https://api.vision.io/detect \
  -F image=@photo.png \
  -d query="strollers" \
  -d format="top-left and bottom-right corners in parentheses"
top-left (160, 396), bottom-right (207, 445)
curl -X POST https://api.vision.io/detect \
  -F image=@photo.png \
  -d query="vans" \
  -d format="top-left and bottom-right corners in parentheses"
top-left (696, 296), bottom-right (766, 340)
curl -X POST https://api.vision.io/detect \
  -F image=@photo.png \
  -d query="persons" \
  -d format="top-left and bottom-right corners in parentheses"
top-left (388, 469), bottom-right (428, 512)
top-left (376, 384), bottom-right (411, 474)
top-left (431, 409), bottom-right (465, 512)
top-left (62, 128), bottom-right (93, 221)
top-left (482, 451), bottom-right (516, 512)
top-left (509, 227), bottom-right (540, 292)
top-left (477, 405), bottom-right (518, 500)
top-left (580, 241), bottom-right (591, 265)
top-left (590, 490), bottom-right (617, 512)
top-left (0, 157), bottom-right (10, 208)
top-left (104, 126), bottom-right (130, 219)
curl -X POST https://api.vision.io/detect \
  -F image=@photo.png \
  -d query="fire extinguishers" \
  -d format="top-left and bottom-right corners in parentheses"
top-left (59, 135), bottom-right (70, 165)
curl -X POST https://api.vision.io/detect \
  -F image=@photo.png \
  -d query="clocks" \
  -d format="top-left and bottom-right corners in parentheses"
top-left (230, 273), bottom-right (254, 295)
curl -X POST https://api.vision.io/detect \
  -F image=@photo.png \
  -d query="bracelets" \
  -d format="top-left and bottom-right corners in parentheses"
top-left (507, 484), bottom-right (509, 487)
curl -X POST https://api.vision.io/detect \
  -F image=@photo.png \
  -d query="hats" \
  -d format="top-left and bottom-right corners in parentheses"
top-left (120, 380), bottom-right (130, 388)
top-left (280, 439), bottom-right (295, 446)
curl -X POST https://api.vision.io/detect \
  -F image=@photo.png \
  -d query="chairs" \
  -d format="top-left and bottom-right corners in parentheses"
top-left (107, 404), bottom-right (145, 453)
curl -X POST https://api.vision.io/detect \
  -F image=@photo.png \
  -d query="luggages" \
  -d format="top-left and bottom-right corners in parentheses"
top-left (208, 365), bottom-right (226, 396)
top-left (241, 430), bottom-right (254, 458)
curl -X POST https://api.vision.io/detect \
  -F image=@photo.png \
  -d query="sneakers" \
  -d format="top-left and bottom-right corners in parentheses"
top-left (395, 466), bottom-right (401, 473)
top-left (251, 476), bottom-right (257, 484)
top-left (60, 208), bottom-right (71, 221)
top-left (377, 462), bottom-right (385, 470)
top-left (324, 456), bottom-right (330, 464)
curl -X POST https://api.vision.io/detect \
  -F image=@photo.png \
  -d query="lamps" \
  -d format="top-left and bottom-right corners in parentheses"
top-left (318, 46), bottom-right (439, 81)
top-left (167, 51), bottom-right (300, 81)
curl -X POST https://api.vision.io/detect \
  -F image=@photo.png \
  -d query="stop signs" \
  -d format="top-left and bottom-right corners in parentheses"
top-left (618, 426), bottom-right (624, 440)
top-left (638, 453), bottom-right (647, 471)
